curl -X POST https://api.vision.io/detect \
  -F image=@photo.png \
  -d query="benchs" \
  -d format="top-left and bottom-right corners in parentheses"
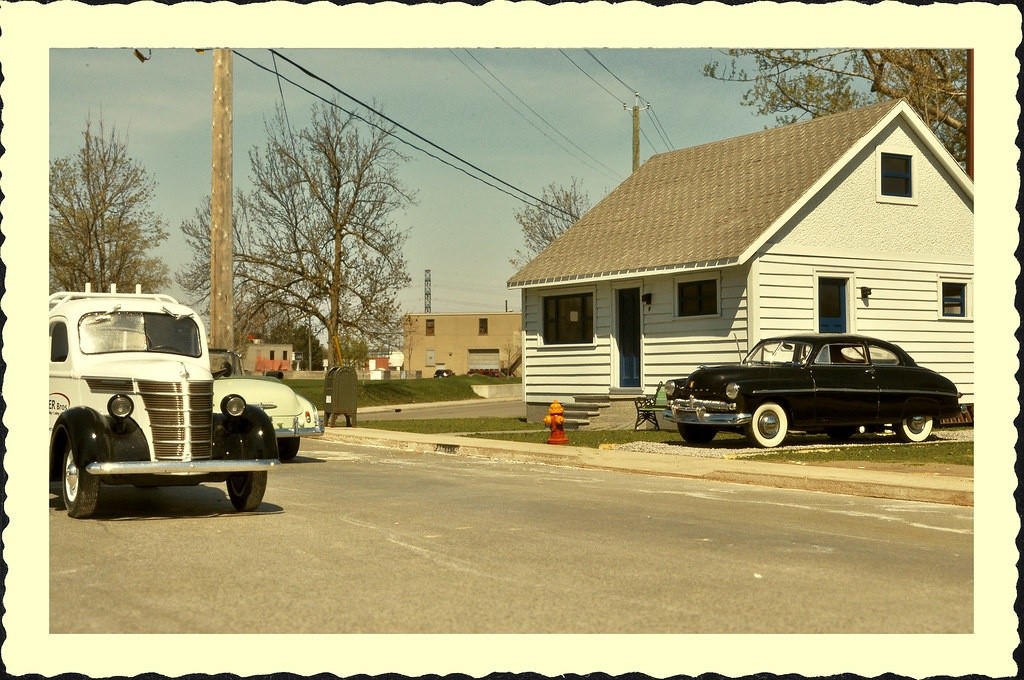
top-left (635, 381), bottom-right (667, 431)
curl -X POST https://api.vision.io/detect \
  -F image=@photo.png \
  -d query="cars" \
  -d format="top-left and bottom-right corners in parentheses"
top-left (209, 347), bottom-right (325, 460)
top-left (662, 334), bottom-right (967, 448)
top-left (434, 370), bottom-right (456, 378)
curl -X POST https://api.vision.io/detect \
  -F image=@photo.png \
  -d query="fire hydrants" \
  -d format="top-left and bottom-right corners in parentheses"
top-left (543, 400), bottom-right (570, 444)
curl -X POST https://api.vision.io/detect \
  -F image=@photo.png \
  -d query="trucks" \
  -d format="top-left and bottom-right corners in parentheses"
top-left (49, 281), bottom-right (281, 520)
